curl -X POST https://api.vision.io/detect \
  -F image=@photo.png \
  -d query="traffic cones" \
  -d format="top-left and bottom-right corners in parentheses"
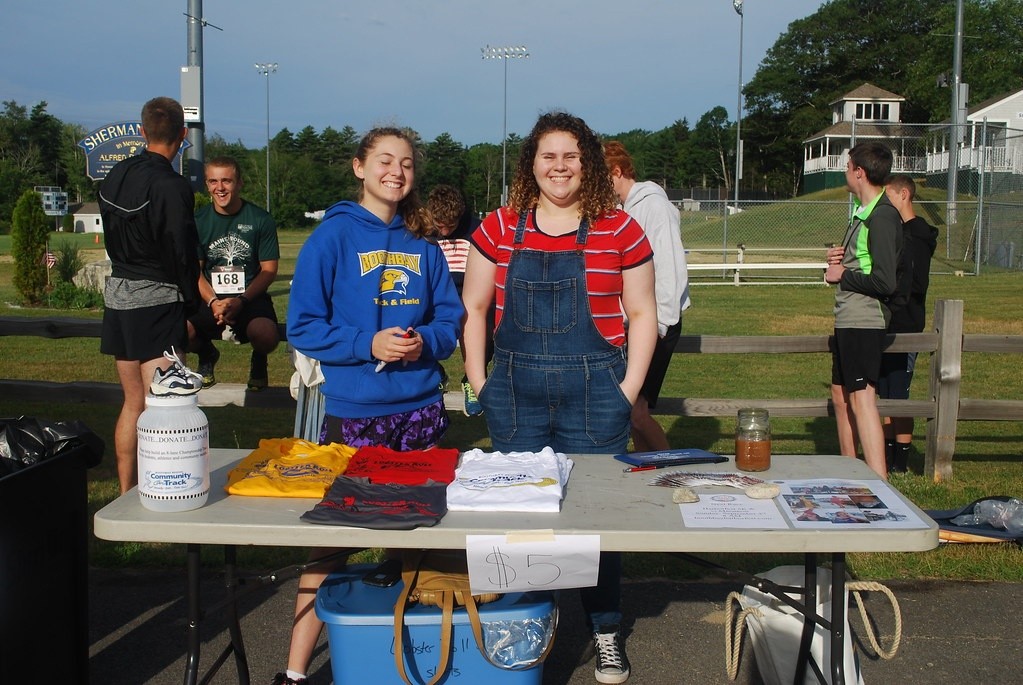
top-left (96, 234), bottom-right (99, 244)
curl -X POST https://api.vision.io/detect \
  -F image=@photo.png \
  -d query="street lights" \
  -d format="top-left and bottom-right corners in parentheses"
top-left (732, 0), bottom-right (746, 205)
top-left (480, 44), bottom-right (530, 208)
top-left (255, 63), bottom-right (278, 213)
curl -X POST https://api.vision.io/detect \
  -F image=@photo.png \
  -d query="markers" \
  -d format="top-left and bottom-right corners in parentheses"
top-left (375, 329), bottom-right (414, 373)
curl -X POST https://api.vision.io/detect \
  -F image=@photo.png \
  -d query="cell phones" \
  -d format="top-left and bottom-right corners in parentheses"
top-left (363, 558), bottom-right (403, 587)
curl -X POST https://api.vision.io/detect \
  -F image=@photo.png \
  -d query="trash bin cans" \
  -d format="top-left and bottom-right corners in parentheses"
top-left (0, 413), bottom-right (90, 685)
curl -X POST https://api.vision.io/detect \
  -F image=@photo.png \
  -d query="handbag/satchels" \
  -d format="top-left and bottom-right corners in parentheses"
top-left (725, 562), bottom-right (901, 685)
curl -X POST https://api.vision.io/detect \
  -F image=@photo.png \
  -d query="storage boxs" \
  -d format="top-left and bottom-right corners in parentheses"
top-left (314, 563), bottom-right (559, 685)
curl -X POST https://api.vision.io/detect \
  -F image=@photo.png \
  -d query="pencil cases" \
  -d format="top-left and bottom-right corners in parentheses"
top-left (614, 448), bottom-right (728, 467)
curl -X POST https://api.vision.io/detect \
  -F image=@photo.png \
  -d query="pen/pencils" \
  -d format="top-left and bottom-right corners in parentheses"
top-left (623, 465), bottom-right (668, 472)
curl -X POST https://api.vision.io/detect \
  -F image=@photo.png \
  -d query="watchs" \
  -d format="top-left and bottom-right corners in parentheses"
top-left (237, 295), bottom-right (249, 309)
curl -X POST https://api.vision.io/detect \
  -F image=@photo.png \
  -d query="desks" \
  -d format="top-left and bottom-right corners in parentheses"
top-left (93, 447), bottom-right (939, 685)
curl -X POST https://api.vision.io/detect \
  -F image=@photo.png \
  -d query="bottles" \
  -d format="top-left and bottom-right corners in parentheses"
top-left (137, 392), bottom-right (211, 514)
top-left (736, 407), bottom-right (772, 473)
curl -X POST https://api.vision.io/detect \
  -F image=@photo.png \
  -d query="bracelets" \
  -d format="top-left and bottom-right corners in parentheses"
top-left (206, 297), bottom-right (218, 306)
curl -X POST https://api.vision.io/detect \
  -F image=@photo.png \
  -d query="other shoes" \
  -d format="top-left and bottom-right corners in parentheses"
top-left (270, 672), bottom-right (307, 685)
top-left (892, 456), bottom-right (906, 469)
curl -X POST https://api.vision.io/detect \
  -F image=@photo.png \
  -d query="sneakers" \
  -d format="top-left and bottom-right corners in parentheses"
top-left (462, 382), bottom-right (484, 417)
top-left (195, 343), bottom-right (221, 388)
top-left (247, 350), bottom-right (268, 391)
top-left (593, 623), bottom-right (631, 683)
top-left (150, 345), bottom-right (204, 395)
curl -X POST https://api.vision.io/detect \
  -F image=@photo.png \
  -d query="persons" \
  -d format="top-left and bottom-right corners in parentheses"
top-left (101, 96), bottom-right (202, 500)
top-left (459, 112), bottom-right (658, 684)
top-left (269, 127), bottom-right (466, 685)
top-left (875, 174), bottom-right (940, 479)
top-left (423, 184), bottom-right (495, 418)
top-left (826, 140), bottom-right (903, 484)
top-left (186, 156), bottom-right (280, 391)
top-left (600, 139), bottom-right (691, 411)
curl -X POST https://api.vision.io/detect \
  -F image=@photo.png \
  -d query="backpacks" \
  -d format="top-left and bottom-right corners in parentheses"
top-left (393, 550), bottom-right (560, 685)
